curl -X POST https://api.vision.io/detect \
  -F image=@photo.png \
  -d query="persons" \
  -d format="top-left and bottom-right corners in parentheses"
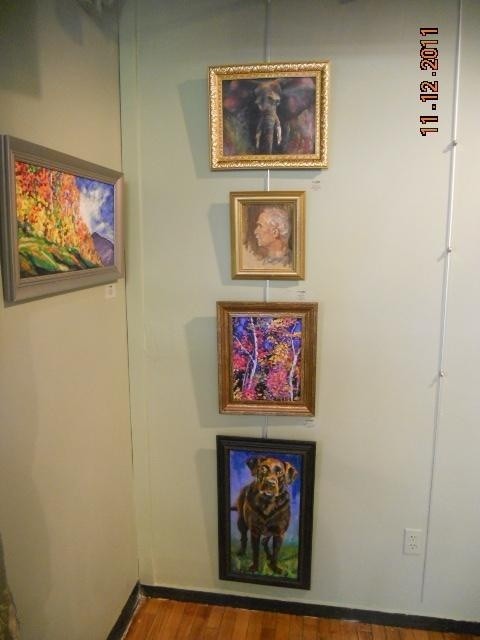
top-left (254, 206), bottom-right (293, 268)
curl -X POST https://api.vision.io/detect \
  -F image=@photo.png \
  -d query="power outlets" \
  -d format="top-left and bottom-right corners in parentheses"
top-left (402, 528), bottom-right (423, 556)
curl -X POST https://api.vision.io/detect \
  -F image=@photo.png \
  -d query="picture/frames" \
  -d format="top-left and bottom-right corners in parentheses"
top-left (216, 435), bottom-right (316, 590)
top-left (216, 300), bottom-right (318, 417)
top-left (0, 134), bottom-right (124, 304)
top-left (208, 61), bottom-right (330, 170)
top-left (229, 189), bottom-right (306, 281)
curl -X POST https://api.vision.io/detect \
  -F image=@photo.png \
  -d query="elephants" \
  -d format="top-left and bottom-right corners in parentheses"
top-left (221, 75), bottom-right (317, 155)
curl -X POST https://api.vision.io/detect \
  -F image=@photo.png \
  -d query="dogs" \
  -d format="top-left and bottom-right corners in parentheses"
top-left (229, 453), bottom-right (300, 576)
